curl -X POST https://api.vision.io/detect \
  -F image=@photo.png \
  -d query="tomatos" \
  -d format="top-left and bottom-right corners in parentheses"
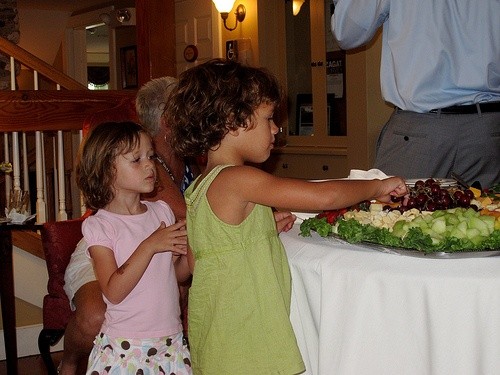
top-left (316, 208), bottom-right (350, 224)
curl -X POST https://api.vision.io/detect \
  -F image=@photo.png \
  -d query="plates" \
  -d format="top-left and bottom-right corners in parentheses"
top-left (310, 227), bottom-right (500, 259)
top-left (404, 178), bottom-right (457, 188)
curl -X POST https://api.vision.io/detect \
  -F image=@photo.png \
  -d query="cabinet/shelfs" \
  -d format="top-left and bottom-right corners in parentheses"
top-left (261, 155), bottom-right (348, 179)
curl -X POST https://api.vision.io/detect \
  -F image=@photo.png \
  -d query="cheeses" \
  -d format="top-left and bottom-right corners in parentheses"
top-left (343, 203), bottom-right (434, 232)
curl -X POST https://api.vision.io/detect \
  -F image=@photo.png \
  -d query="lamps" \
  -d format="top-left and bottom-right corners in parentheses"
top-left (100, 8), bottom-right (137, 28)
top-left (213, 0), bottom-right (246, 31)
top-left (293, 0), bottom-right (305, 16)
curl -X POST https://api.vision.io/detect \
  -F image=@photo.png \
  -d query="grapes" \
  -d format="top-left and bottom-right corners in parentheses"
top-left (359, 178), bottom-right (478, 214)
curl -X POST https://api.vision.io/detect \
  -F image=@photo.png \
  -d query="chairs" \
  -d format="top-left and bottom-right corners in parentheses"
top-left (37, 217), bottom-right (197, 375)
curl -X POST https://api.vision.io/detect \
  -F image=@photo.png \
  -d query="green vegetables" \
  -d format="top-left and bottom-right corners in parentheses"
top-left (298, 214), bottom-right (500, 252)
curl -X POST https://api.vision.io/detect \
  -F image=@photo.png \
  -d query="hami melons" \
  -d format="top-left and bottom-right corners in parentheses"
top-left (469, 187), bottom-right (500, 217)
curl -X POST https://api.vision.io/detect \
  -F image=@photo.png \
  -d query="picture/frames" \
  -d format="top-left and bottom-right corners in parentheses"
top-left (119, 46), bottom-right (137, 88)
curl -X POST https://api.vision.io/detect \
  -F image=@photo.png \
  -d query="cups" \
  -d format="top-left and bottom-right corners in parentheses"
top-left (9, 189), bottom-right (29, 214)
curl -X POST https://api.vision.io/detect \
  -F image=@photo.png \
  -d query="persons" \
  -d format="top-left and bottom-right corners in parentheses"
top-left (52, 76), bottom-right (208, 375)
top-left (74, 119), bottom-right (196, 375)
top-left (329, 1), bottom-right (500, 194)
top-left (163, 56), bottom-right (409, 375)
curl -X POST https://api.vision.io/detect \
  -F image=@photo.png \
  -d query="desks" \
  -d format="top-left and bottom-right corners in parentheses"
top-left (278, 178), bottom-right (500, 374)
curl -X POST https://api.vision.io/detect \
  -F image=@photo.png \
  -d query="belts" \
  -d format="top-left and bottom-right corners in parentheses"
top-left (397, 100), bottom-right (500, 115)
